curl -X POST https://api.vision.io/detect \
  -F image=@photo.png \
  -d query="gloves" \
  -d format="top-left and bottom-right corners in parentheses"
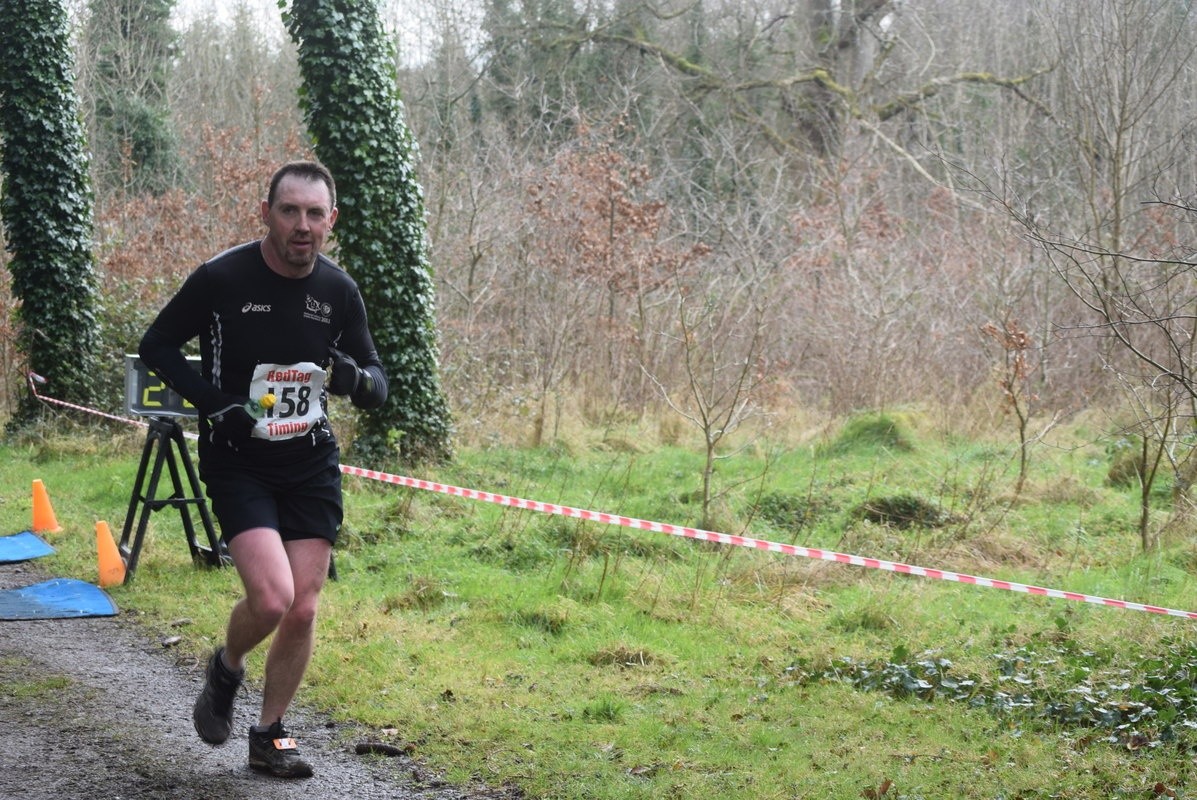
top-left (323, 344), bottom-right (362, 398)
top-left (208, 395), bottom-right (256, 443)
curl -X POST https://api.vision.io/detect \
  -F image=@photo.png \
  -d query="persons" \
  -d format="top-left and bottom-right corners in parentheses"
top-left (139, 162), bottom-right (391, 777)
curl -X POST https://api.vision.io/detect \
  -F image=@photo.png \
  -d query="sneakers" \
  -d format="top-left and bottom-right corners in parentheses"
top-left (192, 646), bottom-right (245, 748)
top-left (248, 717), bottom-right (315, 780)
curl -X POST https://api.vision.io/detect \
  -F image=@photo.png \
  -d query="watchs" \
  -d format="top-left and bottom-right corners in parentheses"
top-left (359, 368), bottom-right (373, 399)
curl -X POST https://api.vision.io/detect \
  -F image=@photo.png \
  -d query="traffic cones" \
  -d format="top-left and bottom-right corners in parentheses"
top-left (32, 479), bottom-right (64, 539)
top-left (95, 520), bottom-right (131, 591)
